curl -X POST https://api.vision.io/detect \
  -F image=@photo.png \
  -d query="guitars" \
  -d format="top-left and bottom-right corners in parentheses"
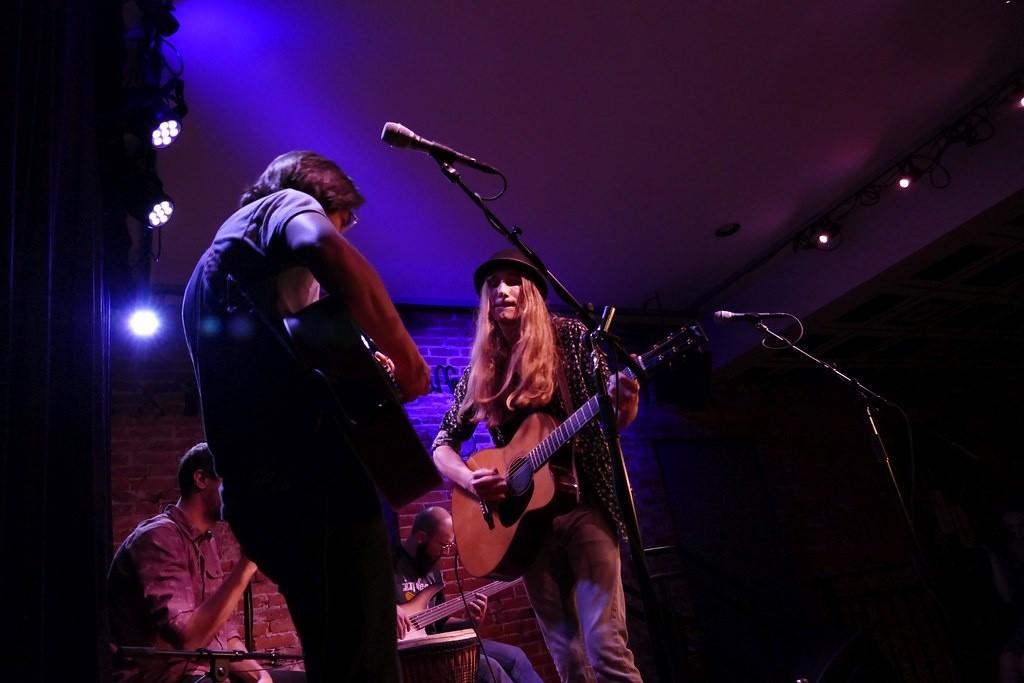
top-left (282, 294), bottom-right (442, 509)
top-left (450, 316), bottom-right (709, 581)
top-left (396, 574), bottom-right (523, 640)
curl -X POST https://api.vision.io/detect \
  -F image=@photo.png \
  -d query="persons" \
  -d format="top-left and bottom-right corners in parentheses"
top-left (387, 507), bottom-right (546, 683)
top-left (103, 442), bottom-right (282, 683)
top-left (181, 149), bottom-right (428, 681)
top-left (429, 249), bottom-right (639, 683)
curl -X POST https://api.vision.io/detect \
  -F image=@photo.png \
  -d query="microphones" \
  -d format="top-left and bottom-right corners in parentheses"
top-left (713, 310), bottom-right (788, 324)
top-left (380, 122), bottom-right (499, 175)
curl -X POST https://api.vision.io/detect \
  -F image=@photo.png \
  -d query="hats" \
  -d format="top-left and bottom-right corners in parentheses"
top-left (473, 248), bottom-right (549, 301)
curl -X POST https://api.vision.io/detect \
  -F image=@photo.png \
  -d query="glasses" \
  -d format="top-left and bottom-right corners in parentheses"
top-left (341, 207), bottom-right (358, 233)
top-left (440, 541), bottom-right (454, 548)
